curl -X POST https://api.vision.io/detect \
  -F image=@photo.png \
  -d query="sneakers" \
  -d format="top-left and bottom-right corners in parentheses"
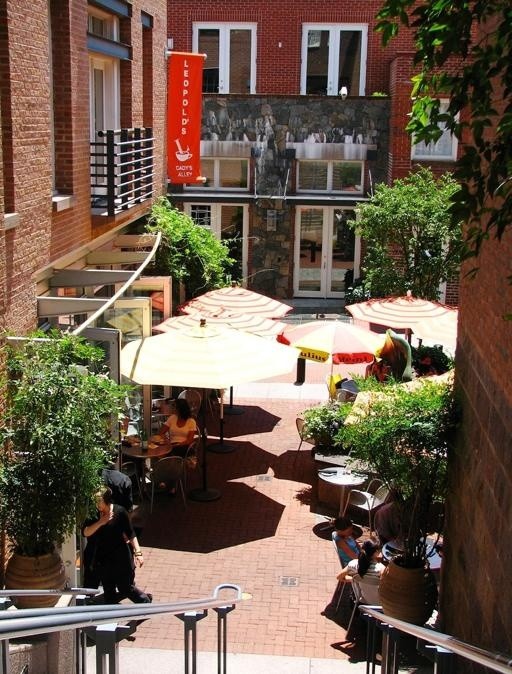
top-left (160, 482), bottom-right (176, 494)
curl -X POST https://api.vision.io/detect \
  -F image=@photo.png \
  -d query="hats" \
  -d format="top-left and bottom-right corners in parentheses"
top-left (337, 380), bottom-right (361, 394)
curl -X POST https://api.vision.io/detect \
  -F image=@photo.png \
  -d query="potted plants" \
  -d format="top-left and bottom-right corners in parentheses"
top-left (0, 328), bottom-right (143, 610)
top-left (295, 349), bottom-right (456, 626)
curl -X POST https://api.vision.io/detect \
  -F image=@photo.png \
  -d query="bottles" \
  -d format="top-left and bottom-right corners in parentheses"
top-left (142, 429), bottom-right (148, 453)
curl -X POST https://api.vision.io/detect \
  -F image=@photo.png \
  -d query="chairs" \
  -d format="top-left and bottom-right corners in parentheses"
top-left (331, 478), bottom-right (392, 639)
top-left (296, 418), bottom-right (320, 455)
top-left (103, 389), bottom-right (217, 514)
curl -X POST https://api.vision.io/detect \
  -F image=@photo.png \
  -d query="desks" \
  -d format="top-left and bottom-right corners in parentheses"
top-left (316, 466), bottom-right (368, 531)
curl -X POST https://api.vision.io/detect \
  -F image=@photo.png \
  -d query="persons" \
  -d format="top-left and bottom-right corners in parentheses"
top-left (156, 397), bottom-right (197, 495)
top-left (330, 515), bottom-right (384, 568)
top-left (414, 351), bottom-right (437, 376)
top-left (335, 539), bottom-right (386, 606)
top-left (80, 483), bottom-right (154, 603)
top-left (89, 449), bottom-right (134, 562)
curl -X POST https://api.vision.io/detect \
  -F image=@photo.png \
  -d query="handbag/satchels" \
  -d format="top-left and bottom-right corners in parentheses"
top-left (84, 566), bottom-right (100, 588)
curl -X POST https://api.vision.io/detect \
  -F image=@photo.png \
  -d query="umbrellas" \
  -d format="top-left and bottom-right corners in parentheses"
top-left (344, 289), bottom-right (457, 344)
top-left (176, 279), bottom-right (293, 408)
top-left (151, 309), bottom-right (286, 444)
top-left (277, 316), bottom-right (386, 400)
top-left (119, 317), bottom-right (303, 492)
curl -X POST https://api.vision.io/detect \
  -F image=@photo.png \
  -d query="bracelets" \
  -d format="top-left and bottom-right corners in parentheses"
top-left (135, 552), bottom-right (142, 556)
